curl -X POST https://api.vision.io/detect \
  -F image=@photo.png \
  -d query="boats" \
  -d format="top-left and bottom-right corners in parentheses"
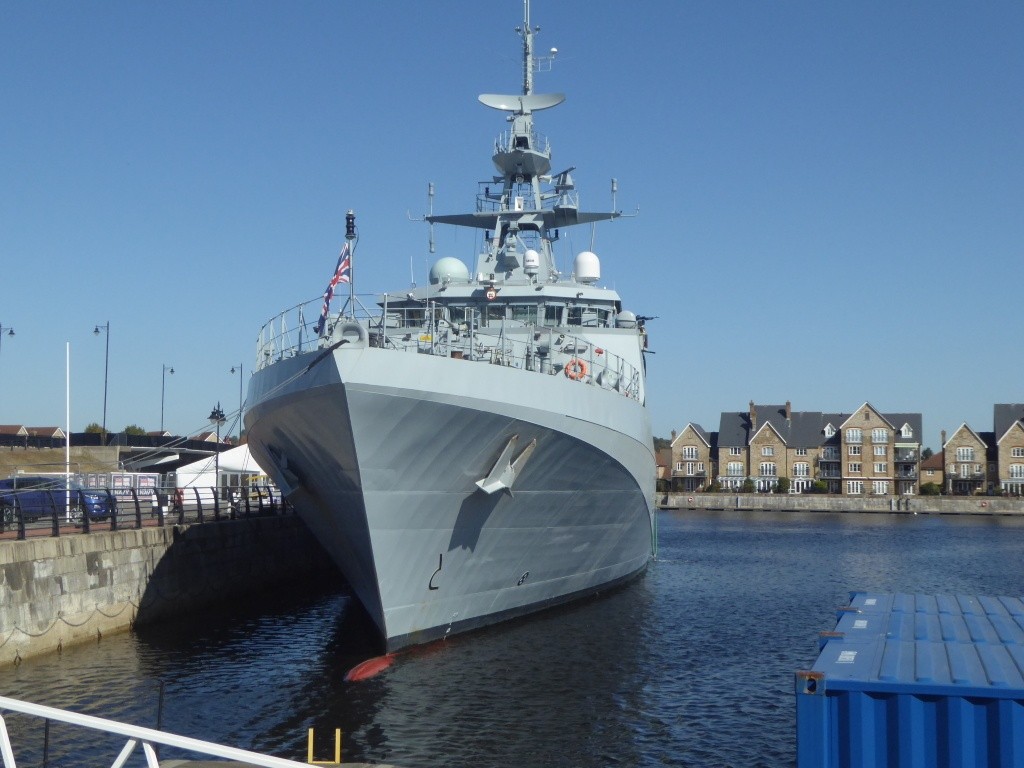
top-left (243, 2), bottom-right (657, 656)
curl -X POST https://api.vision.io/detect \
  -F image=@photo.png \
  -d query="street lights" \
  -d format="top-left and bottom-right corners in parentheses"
top-left (93, 320), bottom-right (109, 446)
top-left (161, 364), bottom-right (174, 446)
top-left (231, 363), bottom-right (242, 445)
top-left (207, 400), bottom-right (226, 513)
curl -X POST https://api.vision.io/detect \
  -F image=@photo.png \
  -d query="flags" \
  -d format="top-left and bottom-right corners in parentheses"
top-left (318, 241), bottom-right (352, 337)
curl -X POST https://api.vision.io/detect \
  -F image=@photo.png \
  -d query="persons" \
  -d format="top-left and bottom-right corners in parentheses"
top-left (233, 490), bottom-right (241, 512)
top-left (173, 489), bottom-right (184, 513)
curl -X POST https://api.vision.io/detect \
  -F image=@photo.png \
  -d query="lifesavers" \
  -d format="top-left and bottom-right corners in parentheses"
top-left (565, 360), bottom-right (586, 379)
top-left (981, 501), bottom-right (987, 507)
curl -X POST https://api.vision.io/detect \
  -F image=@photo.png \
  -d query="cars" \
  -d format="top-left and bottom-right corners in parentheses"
top-left (0, 477), bottom-right (117, 525)
